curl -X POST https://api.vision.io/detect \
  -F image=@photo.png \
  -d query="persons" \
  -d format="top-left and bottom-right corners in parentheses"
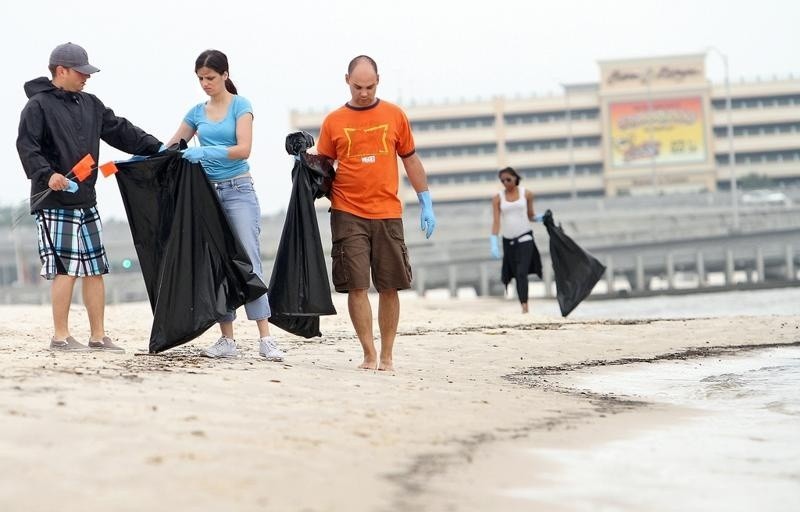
top-left (293, 53), bottom-right (437, 373)
top-left (490, 166), bottom-right (545, 314)
top-left (15, 42), bottom-right (163, 355)
top-left (128, 48), bottom-right (288, 362)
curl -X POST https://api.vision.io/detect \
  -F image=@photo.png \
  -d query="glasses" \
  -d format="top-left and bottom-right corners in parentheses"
top-left (501, 178), bottom-right (512, 183)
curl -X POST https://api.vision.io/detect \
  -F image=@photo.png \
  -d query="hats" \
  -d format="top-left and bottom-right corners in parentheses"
top-left (49, 41), bottom-right (100, 75)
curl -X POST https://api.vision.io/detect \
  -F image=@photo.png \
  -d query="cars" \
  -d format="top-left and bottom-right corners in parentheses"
top-left (740, 188), bottom-right (787, 207)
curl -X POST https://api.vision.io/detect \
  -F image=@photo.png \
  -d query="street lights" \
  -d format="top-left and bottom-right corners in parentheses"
top-left (706, 43), bottom-right (741, 231)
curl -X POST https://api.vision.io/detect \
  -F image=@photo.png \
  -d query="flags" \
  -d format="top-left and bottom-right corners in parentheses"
top-left (101, 159), bottom-right (119, 178)
top-left (75, 166), bottom-right (93, 184)
top-left (74, 153), bottom-right (94, 172)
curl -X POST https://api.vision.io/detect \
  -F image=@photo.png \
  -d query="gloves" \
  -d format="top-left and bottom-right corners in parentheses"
top-left (180, 146), bottom-right (227, 164)
top-left (489, 235), bottom-right (498, 258)
top-left (417, 190), bottom-right (436, 238)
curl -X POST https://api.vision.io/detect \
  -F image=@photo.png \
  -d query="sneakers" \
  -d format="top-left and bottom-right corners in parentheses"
top-left (257, 336), bottom-right (287, 362)
top-left (49, 336), bottom-right (91, 351)
top-left (200, 336), bottom-right (238, 358)
top-left (88, 336), bottom-right (125, 354)
